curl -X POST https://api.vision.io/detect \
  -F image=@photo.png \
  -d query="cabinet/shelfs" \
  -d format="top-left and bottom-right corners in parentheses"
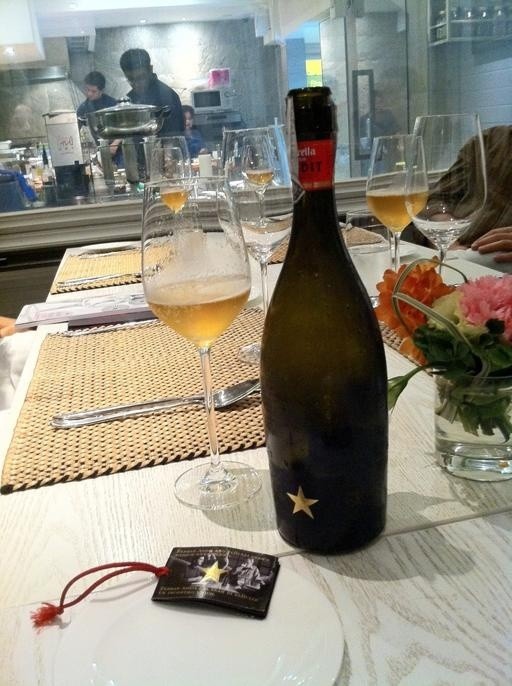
top-left (426, 1), bottom-right (512, 48)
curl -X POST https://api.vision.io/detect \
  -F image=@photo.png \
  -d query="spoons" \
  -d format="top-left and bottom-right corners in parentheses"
top-left (50, 377), bottom-right (259, 428)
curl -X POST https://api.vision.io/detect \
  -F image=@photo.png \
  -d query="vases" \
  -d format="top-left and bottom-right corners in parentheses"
top-left (431, 365), bottom-right (512, 484)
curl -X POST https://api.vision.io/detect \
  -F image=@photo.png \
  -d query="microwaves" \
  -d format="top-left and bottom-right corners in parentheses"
top-left (190, 88), bottom-right (233, 114)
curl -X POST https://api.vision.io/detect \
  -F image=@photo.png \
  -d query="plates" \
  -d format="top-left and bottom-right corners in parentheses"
top-left (82, 241), bottom-right (136, 252)
top-left (54, 566), bottom-right (351, 685)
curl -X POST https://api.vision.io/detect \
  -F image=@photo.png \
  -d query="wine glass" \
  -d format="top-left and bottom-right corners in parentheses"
top-left (214, 125), bottom-right (295, 367)
top-left (140, 174), bottom-right (269, 513)
top-left (366, 127), bottom-right (430, 269)
top-left (402, 107), bottom-right (491, 284)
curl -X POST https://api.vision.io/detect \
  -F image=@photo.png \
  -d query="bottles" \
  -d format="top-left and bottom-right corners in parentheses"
top-left (258, 85), bottom-right (389, 553)
top-left (434, 3), bottom-right (512, 41)
top-left (40, 148), bottom-right (58, 207)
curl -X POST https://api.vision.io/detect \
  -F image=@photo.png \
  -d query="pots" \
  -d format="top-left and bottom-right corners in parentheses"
top-left (77, 96), bottom-right (173, 139)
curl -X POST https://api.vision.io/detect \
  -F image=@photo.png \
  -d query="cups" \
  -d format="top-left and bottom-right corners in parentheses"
top-left (143, 135), bottom-right (195, 215)
top-left (342, 209), bottom-right (391, 310)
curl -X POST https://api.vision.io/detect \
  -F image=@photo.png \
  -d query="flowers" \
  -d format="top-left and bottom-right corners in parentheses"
top-left (367, 248), bottom-right (512, 448)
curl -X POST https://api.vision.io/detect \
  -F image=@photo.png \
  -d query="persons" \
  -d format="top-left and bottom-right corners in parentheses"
top-left (400, 123), bottom-right (512, 264)
top-left (172, 549), bottom-right (273, 591)
top-left (120, 48), bottom-right (184, 169)
top-left (182, 104), bottom-right (210, 164)
top-left (76, 71), bottom-right (119, 131)
